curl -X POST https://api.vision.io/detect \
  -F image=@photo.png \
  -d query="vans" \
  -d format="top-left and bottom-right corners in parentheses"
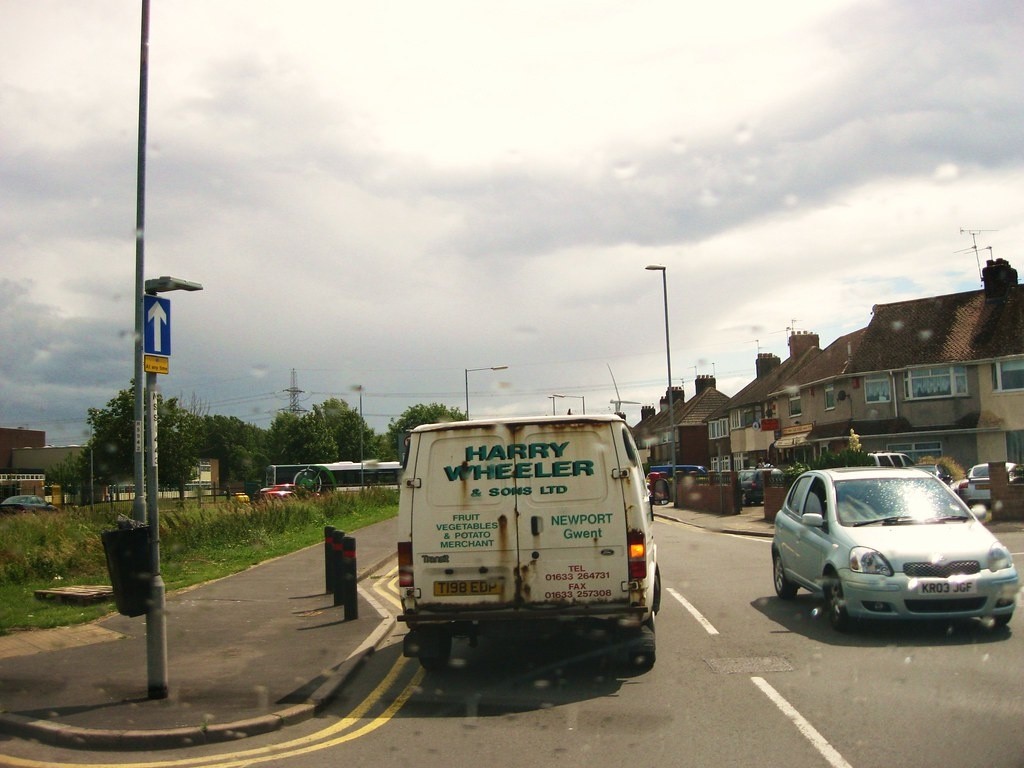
top-left (395, 416), bottom-right (661, 673)
top-left (650, 465), bottom-right (709, 502)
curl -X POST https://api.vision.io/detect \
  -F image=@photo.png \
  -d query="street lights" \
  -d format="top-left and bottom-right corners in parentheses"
top-left (645, 265), bottom-right (677, 503)
top-left (553, 394), bottom-right (586, 415)
top-left (548, 397), bottom-right (555, 417)
top-left (465, 366), bottom-right (508, 420)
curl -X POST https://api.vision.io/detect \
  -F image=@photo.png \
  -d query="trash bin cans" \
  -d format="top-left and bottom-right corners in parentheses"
top-left (101, 525), bottom-right (151, 617)
top-left (245, 480), bottom-right (262, 500)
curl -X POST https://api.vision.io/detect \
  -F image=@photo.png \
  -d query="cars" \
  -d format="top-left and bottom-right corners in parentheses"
top-left (1, 495), bottom-right (58, 515)
top-left (261, 484), bottom-right (319, 499)
top-left (751, 468), bottom-right (785, 506)
top-left (772, 465), bottom-right (1019, 632)
top-left (867, 451), bottom-right (915, 468)
top-left (957, 462), bottom-right (1018, 509)
top-left (914, 464), bottom-right (955, 490)
top-left (738, 469), bottom-right (755, 506)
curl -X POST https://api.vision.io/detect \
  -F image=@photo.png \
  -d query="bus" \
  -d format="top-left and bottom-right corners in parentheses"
top-left (318, 461), bottom-right (403, 496)
top-left (265, 461), bottom-right (353, 486)
